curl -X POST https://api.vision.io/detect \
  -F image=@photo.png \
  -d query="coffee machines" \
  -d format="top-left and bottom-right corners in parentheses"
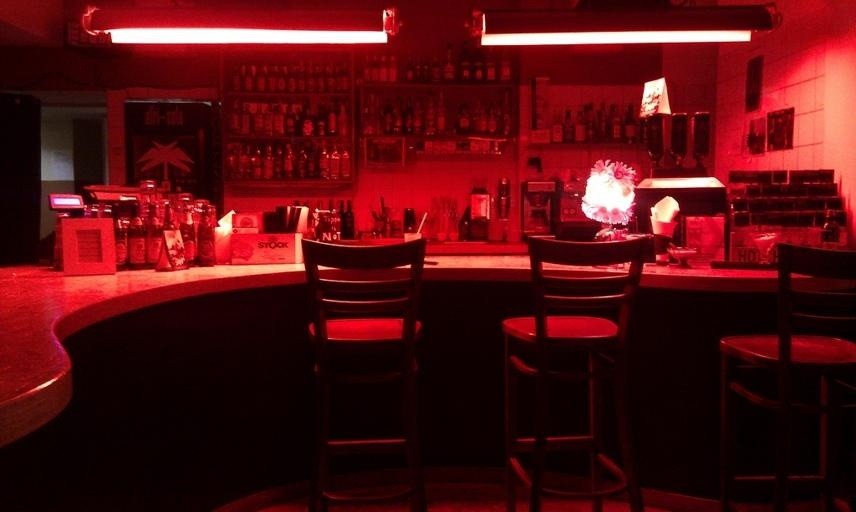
top-left (521, 181), bottom-right (556, 241)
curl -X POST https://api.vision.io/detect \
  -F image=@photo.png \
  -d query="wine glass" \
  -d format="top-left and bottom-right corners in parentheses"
top-left (667, 247), bottom-right (697, 266)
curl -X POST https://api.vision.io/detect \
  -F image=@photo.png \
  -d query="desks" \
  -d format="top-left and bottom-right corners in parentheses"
top-left (0, 254), bottom-right (856, 444)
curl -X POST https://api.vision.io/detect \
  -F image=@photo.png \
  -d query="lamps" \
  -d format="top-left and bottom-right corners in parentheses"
top-left (463, 0), bottom-right (783, 46)
top-left (83, 0), bottom-right (401, 46)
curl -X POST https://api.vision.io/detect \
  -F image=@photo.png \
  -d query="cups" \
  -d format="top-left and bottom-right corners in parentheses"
top-left (405, 233), bottom-right (421, 267)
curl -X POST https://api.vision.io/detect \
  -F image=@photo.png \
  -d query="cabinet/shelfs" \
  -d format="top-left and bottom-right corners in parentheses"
top-left (355, 56), bottom-right (520, 168)
top-left (222, 52), bottom-right (356, 188)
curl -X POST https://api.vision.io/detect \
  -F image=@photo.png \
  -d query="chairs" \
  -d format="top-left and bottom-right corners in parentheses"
top-left (717, 243), bottom-right (856, 512)
top-left (500, 237), bottom-right (649, 512)
top-left (302, 238), bottom-right (426, 512)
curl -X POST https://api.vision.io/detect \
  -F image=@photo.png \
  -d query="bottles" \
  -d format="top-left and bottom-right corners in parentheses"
top-left (403, 206), bottom-right (461, 241)
top-left (113, 195), bottom-right (216, 269)
top-left (224, 50), bottom-right (515, 187)
top-left (497, 176), bottom-right (509, 220)
top-left (563, 102), bottom-right (650, 144)
top-left (821, 210), bottom-right (841, 243)
top-left (291, 199), bottom-right (355, 240)
